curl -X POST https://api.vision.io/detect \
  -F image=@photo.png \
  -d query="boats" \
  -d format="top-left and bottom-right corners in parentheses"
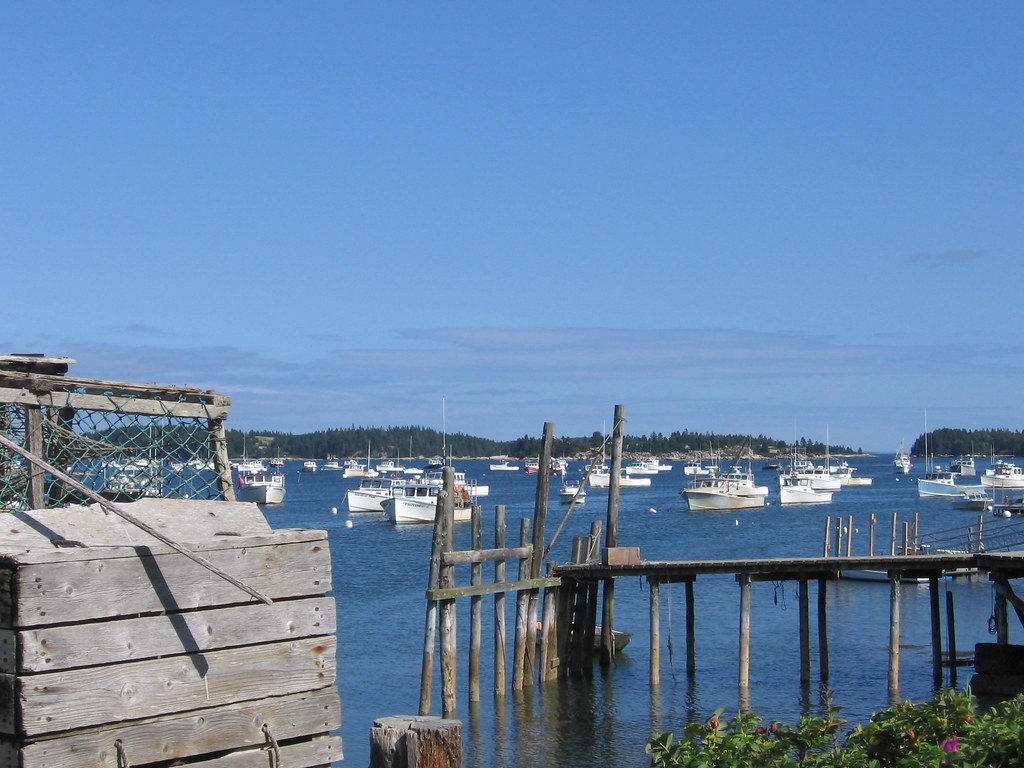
top-left (319, 395), bottom-right (490, 525)
top-left (488, 459), bottom-right (520, 471)
top-left (991, 494), bottom-right (1024, 517)
top-left (950, 440), bottom-right (978, 476)
top-left (583, 419), bottom-right (673, 488)
top-left (892, 437), bottom-right (913, 474)
top-left (557, 478), bottom-right (588, 505)
top-left (522, 449), bottom-right (569, 477)
top-left (98, 417), bottom-right (287, 505)
top-left (301, 460), bottom-right (318, 472)
top-left (951, 489), bottom-right (993, 511)
top-left (981, 441), bottom-right (1024, 488)
top-left (934, 546), bottom-right (978, 577)
top-left (838, 542), bottom-right (930, 583)
top-left (536, 618), bottom-right (632, 653)
top-left (678, 416), bottom-right (876, 512)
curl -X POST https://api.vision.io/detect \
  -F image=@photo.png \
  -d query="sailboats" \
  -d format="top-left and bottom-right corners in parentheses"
top-left (916, 408), bottom-right (989, 498)
